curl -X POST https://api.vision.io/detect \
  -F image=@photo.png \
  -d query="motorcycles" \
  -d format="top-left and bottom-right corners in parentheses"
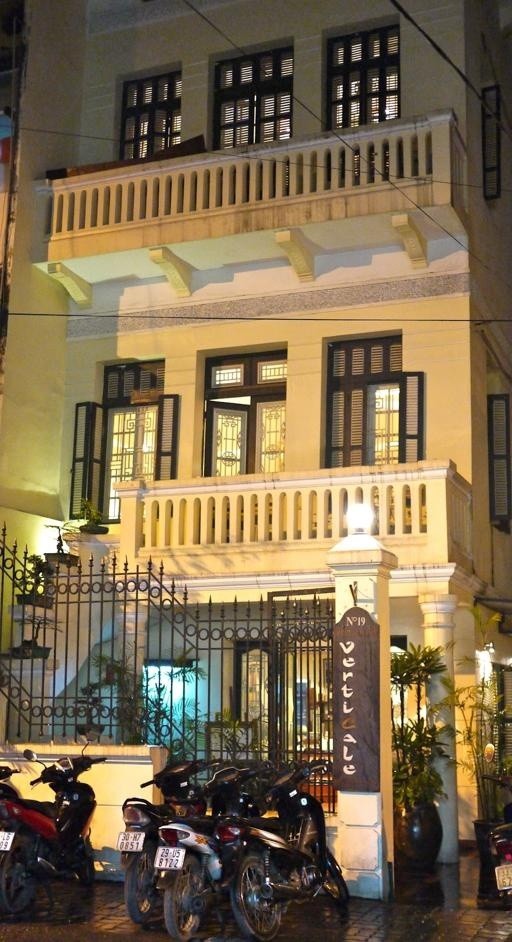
top-left (0, 719), bottom-right (115, 919)
top-left (480, 739), bottom-right (512, 913)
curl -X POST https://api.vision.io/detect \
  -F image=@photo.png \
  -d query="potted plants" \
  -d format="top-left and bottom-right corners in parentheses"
top-left (391, 642), bottom-right (457, 875)
top-left (8, 498), bottom-right (134, 741)
top-left (434, 677), bottom-right (512, 910)
